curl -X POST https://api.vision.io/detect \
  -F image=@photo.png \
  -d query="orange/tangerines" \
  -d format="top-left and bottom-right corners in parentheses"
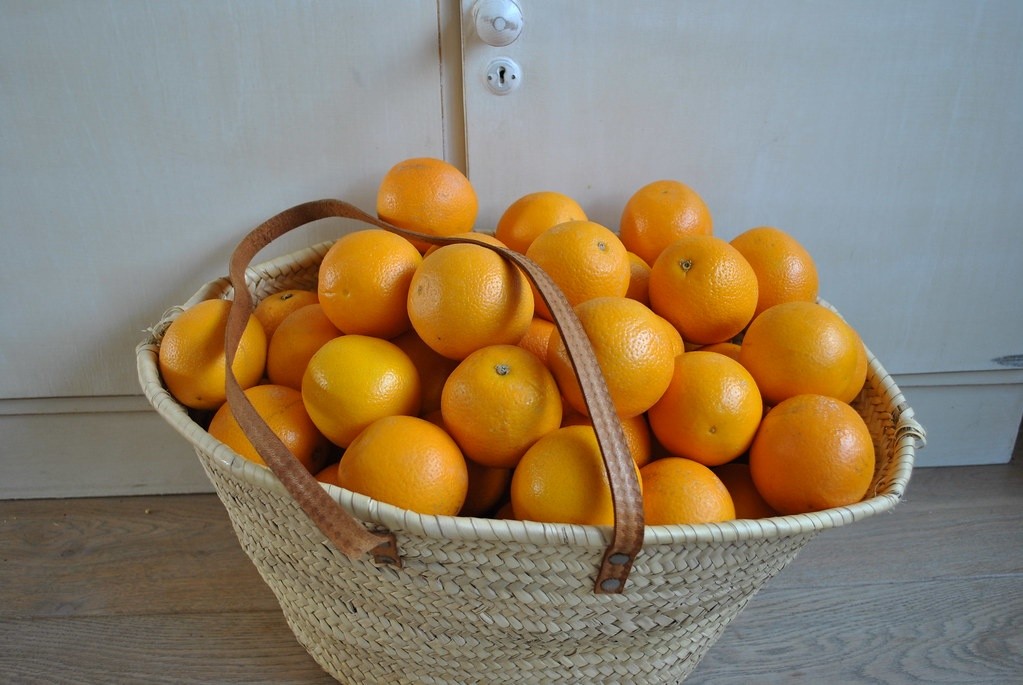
top-left (158, 158), bottom-right (877, 527)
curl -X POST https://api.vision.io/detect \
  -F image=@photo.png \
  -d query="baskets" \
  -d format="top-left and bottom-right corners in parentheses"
top-left (134, 200), bottom-right (926, 684)
